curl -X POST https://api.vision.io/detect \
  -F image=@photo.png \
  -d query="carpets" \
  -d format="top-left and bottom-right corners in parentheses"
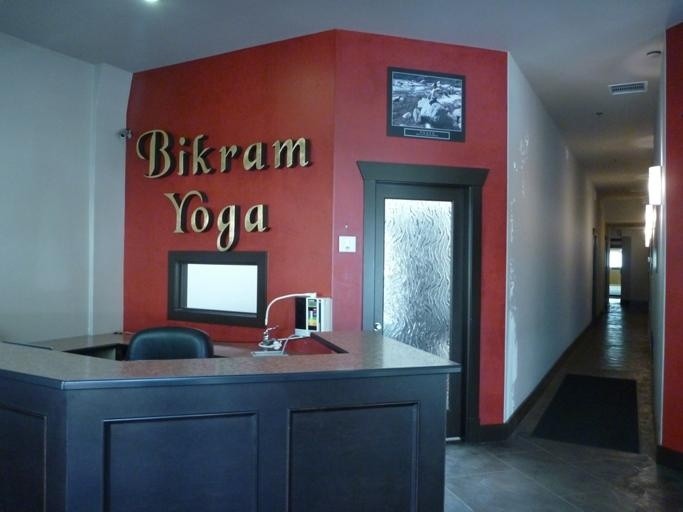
top-left (530, 373), bottom-right (640, 454)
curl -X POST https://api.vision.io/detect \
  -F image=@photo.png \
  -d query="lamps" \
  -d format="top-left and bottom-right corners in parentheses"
top-left (646, 51), bottom-right (661, 58)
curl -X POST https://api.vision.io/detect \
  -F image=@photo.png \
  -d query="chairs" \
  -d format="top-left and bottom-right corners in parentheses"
top-left (127, 326), bottom-right (213, 361)
